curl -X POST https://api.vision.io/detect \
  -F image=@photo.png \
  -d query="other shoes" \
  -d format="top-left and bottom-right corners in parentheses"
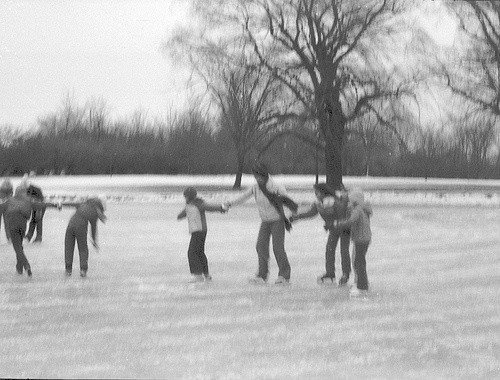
top-left (80, 269), bottom-right (87, 277)
top-left (25, 266), bottom-right (32, 277)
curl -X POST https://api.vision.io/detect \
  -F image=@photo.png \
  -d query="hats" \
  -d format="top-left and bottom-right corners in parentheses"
top-left (347, 190), bottom-right (365, 205)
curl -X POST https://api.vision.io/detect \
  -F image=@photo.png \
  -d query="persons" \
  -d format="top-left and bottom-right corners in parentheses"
top-left (0, 180), bottom-right (107, 278)
top-left (177, 186), bottom-right (227, 281)
top-left (289, 183), bottom-right (373, 298)
top-left (223, 162), bottom-right (298, 285)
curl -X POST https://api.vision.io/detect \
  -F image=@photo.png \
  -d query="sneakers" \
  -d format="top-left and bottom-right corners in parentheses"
top-left (348, 287), bottom-right (369, 301)
top-left (317, 272), bottom-right (336, 285)
top-left (248, 275), bottom-right (267, 285)
top-left (275, 275), bottom-right (291, 287)
top-left (338, 273), bottom-right (350, 288)
